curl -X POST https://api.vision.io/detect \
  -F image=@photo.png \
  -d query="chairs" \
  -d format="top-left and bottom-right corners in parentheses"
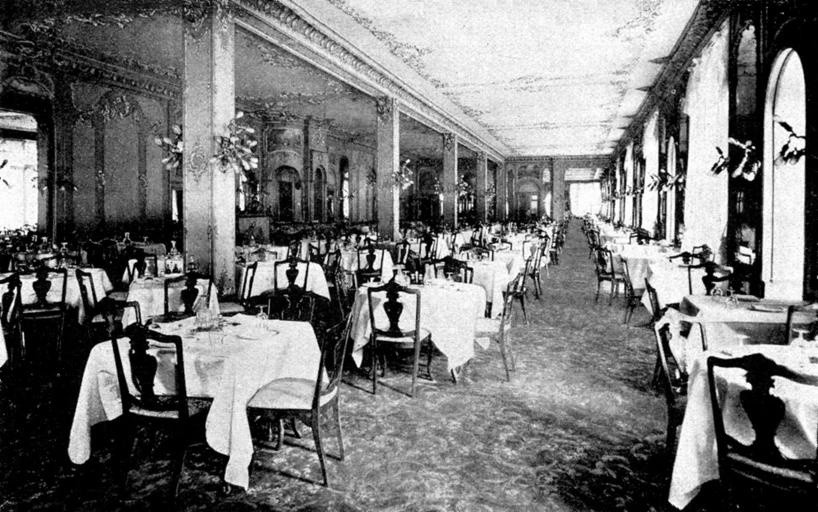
top-left (234, 214), bottom-right (569, 397)
top-left (0, 213), bottom-right (359, 512)
top-left (645, 278), bottom-right (818, 511)
top-left (583, 213), bottom-right (734, 325)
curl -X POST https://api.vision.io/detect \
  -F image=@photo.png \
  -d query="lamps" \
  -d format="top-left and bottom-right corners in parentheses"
top-left (597, 120), bottom-right (813, 202)
top-left (360, 159), bottom-right (415, 196)
top-left (155, 110), bottom-right (263, 184)
top-left (431, 173), bottom-right (469, 202)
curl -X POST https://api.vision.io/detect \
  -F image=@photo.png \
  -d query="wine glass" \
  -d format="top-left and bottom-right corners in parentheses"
top-left (1, 231), bottom-right (529, 357)
top-left (640, 234), bottom-right (818, 373)
